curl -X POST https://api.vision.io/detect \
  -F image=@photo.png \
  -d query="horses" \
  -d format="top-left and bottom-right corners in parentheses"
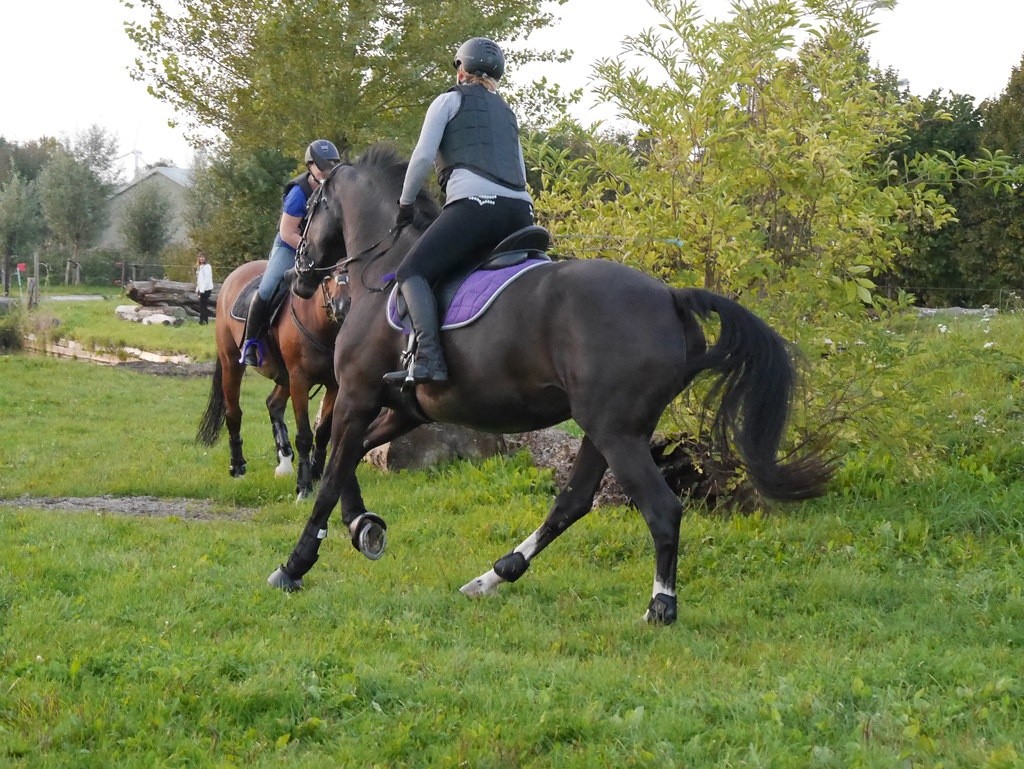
top-left (196, 142), bottom-right (840, 628)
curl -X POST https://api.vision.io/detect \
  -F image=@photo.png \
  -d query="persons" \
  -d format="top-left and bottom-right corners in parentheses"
top-left (381, 37), bottom-right (534, 384)
top-left (240, 139), bottom-right (340, 365)
top-left (194, 253), bottom-right (214, 324)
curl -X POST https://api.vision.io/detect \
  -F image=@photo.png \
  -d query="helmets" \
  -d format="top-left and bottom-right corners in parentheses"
top-left (305, 140), bottom-right (340, 164)
top-left (453, 37), bottom-right (504, 82)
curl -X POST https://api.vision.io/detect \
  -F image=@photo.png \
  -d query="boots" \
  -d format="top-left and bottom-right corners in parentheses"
top-left (240, 290), bottom-right (273, 366)
top-left (382, 274), bottom-right (448, 381)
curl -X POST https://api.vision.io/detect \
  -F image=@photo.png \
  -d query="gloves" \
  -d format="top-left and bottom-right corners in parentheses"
top-left (396, 206), bottom-right (414, 228)
